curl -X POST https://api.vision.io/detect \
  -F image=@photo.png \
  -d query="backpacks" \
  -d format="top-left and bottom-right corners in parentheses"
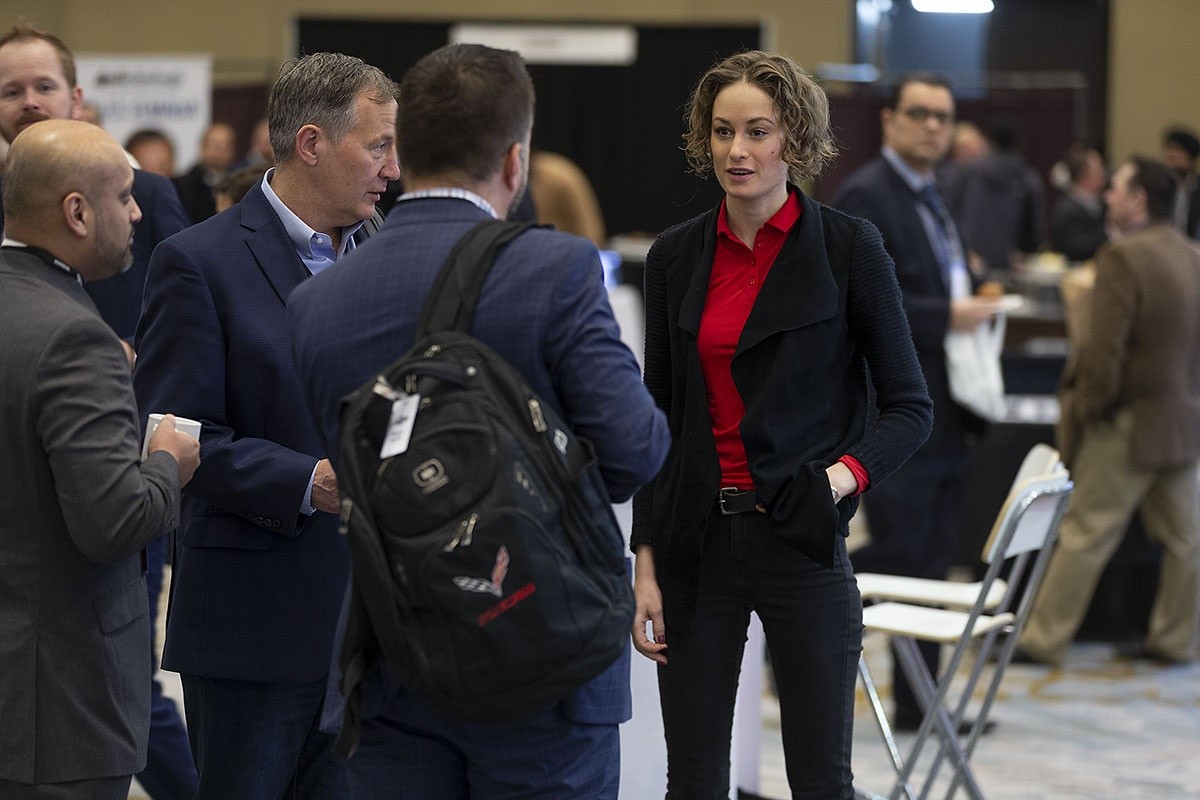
top-left (330, 219), bottom-right (637, 723)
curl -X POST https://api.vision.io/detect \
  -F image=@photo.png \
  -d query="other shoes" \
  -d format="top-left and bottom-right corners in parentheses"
top-left (1115, 633), bottom-right (1176, 661)
top-left (975, 640), bottom-right (1039, 664)
top-left (894, 700), bottom-right (995, 735)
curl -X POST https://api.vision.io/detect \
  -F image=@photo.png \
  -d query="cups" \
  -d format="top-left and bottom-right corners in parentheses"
top-left (140, 413), bottom-right (202, 466)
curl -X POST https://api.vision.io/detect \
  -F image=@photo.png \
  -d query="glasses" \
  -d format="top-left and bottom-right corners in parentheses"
top-left (905, 106), bottom-right (955, 122)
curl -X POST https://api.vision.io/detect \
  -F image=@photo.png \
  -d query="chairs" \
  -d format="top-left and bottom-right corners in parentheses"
top-left (852, 446), bottom-right (1077, 800)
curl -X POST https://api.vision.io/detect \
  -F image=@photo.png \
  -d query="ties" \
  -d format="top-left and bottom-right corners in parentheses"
top-left (919, 184), bottom-right (963, 270)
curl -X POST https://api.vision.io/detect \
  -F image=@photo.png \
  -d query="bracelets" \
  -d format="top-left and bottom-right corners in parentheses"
top-left (831, 486), bottom-right (842, 505)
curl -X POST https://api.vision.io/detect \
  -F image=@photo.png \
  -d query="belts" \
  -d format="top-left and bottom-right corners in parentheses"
top-left (715, 486), bottom-right (763, 515)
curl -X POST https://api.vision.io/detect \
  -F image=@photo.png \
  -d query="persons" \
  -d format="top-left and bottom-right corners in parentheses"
top-left (832, 74), bottom-right (1200, 736)
top-left (0, 26), bottom-right (647, 800)
top-left (631, 50), bottom-right (933, 800)
